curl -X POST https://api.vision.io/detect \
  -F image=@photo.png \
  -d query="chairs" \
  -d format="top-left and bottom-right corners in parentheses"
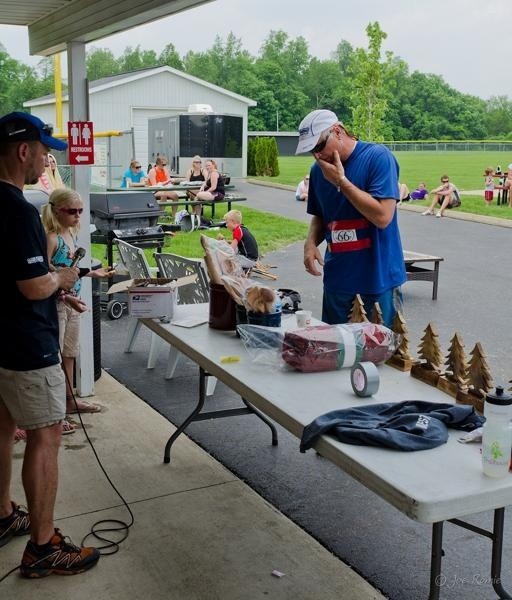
top-left (114, 238), bottom-right (217, 396)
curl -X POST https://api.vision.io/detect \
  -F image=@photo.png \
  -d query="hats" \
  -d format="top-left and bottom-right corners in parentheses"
top-left (0, 112), bottom-right (68, 151)
top-left (295, 109), bottom-right (339, 156)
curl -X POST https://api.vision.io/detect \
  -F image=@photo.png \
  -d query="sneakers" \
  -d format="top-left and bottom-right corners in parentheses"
top-left (421, 209), bottom-right (434, 215)
top-left (436, 211), bottom-right (441, 218)
top-left (0, 502), bottom-right (31, 548)
top-left (20, 528), bottom-right (100, 578)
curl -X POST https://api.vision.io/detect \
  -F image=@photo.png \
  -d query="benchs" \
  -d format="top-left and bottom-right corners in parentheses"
top-left (155, 195), bottom-right (246, 219)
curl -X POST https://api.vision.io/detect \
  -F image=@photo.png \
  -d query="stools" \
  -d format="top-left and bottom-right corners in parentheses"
top-left (494, 187), bottom-right (509, 205)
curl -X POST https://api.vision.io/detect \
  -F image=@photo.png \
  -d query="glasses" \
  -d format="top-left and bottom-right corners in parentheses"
top-left (309, 130), bottom-right (332, 154)
top-left (443, 181), bottom-right (448, 183)
top-left (9, 124), bottom-right (53, 137)
top-left (59, 208), bottom-right (83, 215)
top-left (136, 166), bottom-right (141, 169)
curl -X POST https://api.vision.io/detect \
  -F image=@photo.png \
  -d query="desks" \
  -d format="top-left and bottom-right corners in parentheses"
top-left (483, 174), bottom-right (508, 204)
top-left (107, 185), bottom-right (235, 194)
top-left (138, 302), bottom-right (512, 600)
top-left (403, 250), bottom-right (444, 300)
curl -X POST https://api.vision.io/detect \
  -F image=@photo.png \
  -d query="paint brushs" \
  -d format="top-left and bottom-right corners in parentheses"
top-left (200, 235), bottom-right (275, 313)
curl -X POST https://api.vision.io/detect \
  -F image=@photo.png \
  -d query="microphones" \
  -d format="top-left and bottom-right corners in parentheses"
top-left (70, 248), bottom-right (85, 267)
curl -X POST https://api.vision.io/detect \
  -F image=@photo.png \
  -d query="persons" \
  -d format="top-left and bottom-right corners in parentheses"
top-left (503, 164), bottom-right (512, 207)
top-left (1, 110), bottom-right (104, 580)
top-left (33, 153), bottom-right (65, 196)
top-left (121, 159), bottom-right (146, 188)
top-left (38, 186), bottom-right (117, 434)
top-left (193, 159), bottom-right (225, 201)
top-left (217, 209), bottom-right (259, 269)
top-left (484, 167), bottom-right (495, 207)
top-left (186, 155), bottom-right (208, 215)
top-left (396, 182), bottom-right (429, 206)
top-left (292, 109), bottom-right (407, 336)
top-left (295, 175), bottom-right (309, 201)
top-left (420, 174), bottom-right (461, 218)
top-left (148, 157), bottom-right (179, 219)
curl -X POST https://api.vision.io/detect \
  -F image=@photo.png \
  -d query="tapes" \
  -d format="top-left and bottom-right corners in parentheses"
top-left (350, 361), bottom-right (380, 397)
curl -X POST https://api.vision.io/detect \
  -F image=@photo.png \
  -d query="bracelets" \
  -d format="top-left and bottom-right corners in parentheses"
top-left (335, 174), bottom-right (346, 193)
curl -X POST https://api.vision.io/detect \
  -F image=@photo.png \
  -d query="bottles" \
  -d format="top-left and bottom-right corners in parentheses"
top-left (481, 386), bottom-right (512, 482)
top-left (145, 176), bottom-right (149, 187)
top-left (127, 178), bottom-right (130, 188)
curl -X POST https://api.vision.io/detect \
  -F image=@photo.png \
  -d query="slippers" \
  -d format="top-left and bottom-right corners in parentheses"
top-left (66, 401), bottom-right (101, 414)
top-left (61, 421), bottom-right (75, 435)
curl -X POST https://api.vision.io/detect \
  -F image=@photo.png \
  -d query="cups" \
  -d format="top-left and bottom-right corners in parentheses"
top-left (294, 309), bottom-right (313, 328)
